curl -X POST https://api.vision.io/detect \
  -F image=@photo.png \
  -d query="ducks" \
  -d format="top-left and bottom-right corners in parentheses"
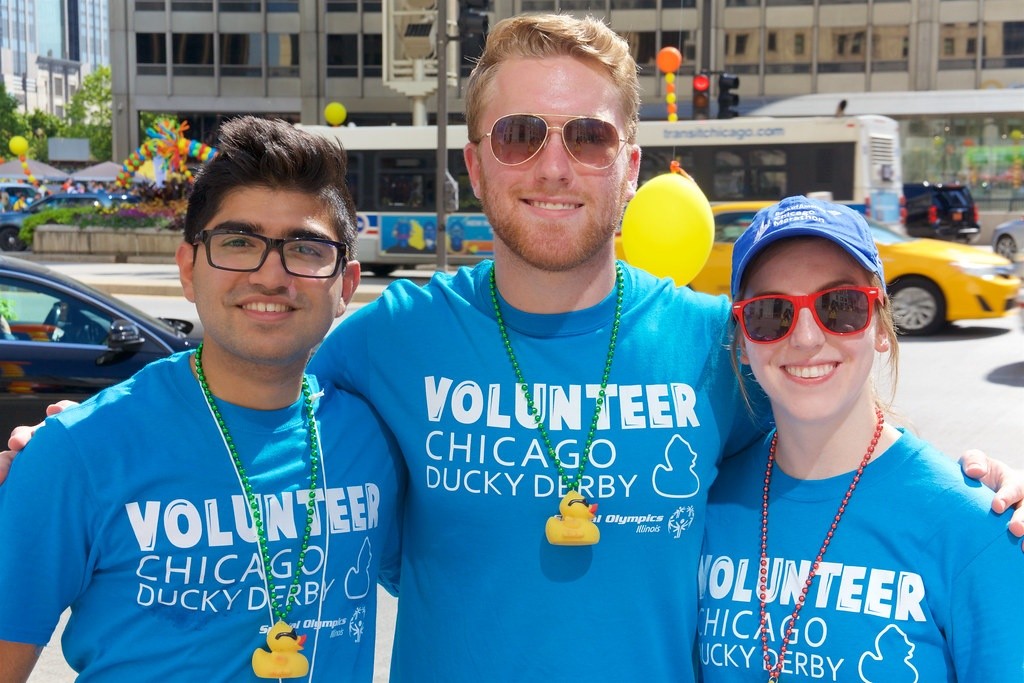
top-left (252, 620), bottom-right (310, 679)
top-left (545, 490), bottom-right (600, 546)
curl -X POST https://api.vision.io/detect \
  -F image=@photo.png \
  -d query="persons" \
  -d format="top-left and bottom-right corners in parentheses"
top-left (0, 177), bottom-right (195, 212)
top-left (692, 193), bottom-right (1022, 681)
top-left (7, 11), bottom-right (1024, 683)
top-left (1, 115), bottom-right (406, 683)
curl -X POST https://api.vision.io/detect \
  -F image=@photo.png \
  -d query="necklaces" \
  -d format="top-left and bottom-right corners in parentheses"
top-left (755, 402), bottom-right (884, 681)
top-left (193, 339), bottom-right (320, 680)
top-left (488, 258), bottom-right (625, 544)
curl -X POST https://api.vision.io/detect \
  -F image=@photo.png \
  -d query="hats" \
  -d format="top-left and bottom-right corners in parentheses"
top-left (731, 195), bottom-right (885, 305)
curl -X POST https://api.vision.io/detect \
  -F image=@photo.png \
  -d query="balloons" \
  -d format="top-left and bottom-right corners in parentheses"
top-left (654, 47), bottom-right (681, 124)
top-left (323, 102), bottom-right (347, 125)
top-left (110, 120), bottom-right (219, 194)
top-left (621, 160), bottom-right (713, 288)
top-left (9, 135), bottom-right (39, 186)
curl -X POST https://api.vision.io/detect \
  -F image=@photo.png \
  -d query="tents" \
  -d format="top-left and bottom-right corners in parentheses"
top-left (0, 158), bottom-right (156, 196)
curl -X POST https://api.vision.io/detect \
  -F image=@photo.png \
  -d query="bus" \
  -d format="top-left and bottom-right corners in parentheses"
top-left (295, 114), bottom-right (906, 277)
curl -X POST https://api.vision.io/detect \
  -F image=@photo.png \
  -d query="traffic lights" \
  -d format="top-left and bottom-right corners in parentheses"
top-left (692, 73), bottom-right (709, 120)
top-left (717, 72), bottom-right (740, 119)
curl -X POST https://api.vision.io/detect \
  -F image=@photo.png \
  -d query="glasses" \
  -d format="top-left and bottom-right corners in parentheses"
top-left (730, 285), bottom-right (885, 345)
top-left (186, 225), bottom-right (347, 280)
top-left (475, 113), bottom-right (631, 170)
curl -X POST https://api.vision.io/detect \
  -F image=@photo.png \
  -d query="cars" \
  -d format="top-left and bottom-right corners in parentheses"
top-left (0, 183), bottom-right (38, 208)
top-left (611, 200), bottom-right (1024, 336)
top-left (991, 217), bottom-right (1024, 282)
top-left (0, 254), bottom-right (203, 452)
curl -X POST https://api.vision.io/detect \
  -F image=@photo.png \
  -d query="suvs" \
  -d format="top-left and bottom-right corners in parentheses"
top-left (904, 183), bottom-right (981, 244)
top-left (0, 194), bottom-right (140, 252)
